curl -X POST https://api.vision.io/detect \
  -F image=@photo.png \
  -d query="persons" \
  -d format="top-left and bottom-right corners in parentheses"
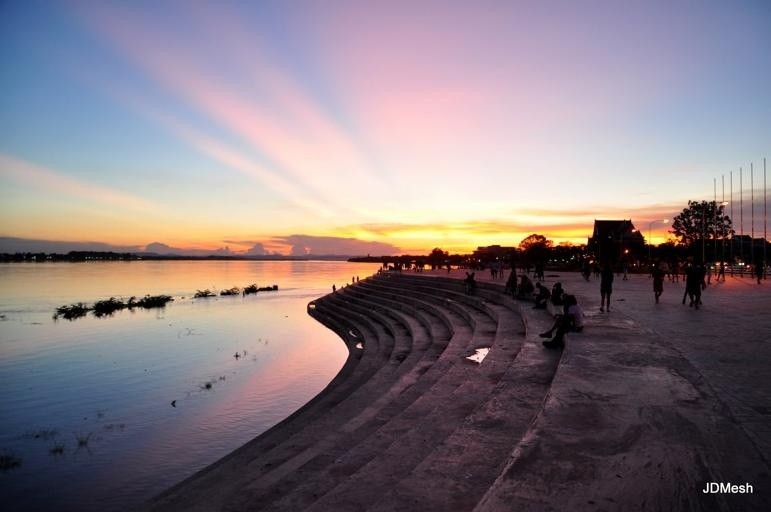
top-left (375, 244), bottom-right (771, 351)
top-left (332, 275), bottom-right (359, 293)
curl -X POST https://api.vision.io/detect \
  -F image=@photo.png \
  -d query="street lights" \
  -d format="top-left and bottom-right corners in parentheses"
top-left (649, 219), bottom-right (670, 266)
top-left (703, 201), bottom-right (729, 263)
top-left (621, 227), bottom-right (637, 262)
